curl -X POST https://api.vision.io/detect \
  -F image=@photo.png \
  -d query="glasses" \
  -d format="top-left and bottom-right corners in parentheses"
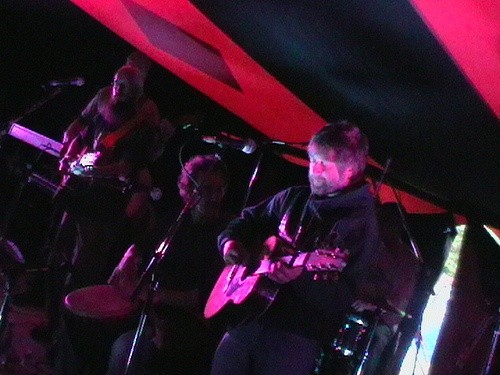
top-left (111, 81), bottom-right (136, 93)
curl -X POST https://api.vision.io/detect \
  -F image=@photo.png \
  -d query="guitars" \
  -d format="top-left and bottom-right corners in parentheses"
top-left (60, 114), bottom-right (202, 178)
top-left (205, 234), bottom-right (350, 324)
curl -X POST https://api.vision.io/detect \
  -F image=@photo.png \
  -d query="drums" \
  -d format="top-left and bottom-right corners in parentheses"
top-left (331, 312), bottom-right (370, 356)
top-left (63, 286), bottom-right (136, 368)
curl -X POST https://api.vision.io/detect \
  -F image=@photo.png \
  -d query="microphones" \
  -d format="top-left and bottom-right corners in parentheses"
top-left (214, 132), bottom-right (257, 154)
top-left (116, 176), bottom-right (164, 201)
top-left (49, 76), bottom-right (86, 86)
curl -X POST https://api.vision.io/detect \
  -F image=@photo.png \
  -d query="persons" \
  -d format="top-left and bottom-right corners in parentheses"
top-left (207, 119), bottom-right (385, 375)
top-left (28, 64), bottom-right (161, 361)
top-left (105, 153), bottom-right (239, 375)
top-left (350, 200), bottom-right (420, 375)
